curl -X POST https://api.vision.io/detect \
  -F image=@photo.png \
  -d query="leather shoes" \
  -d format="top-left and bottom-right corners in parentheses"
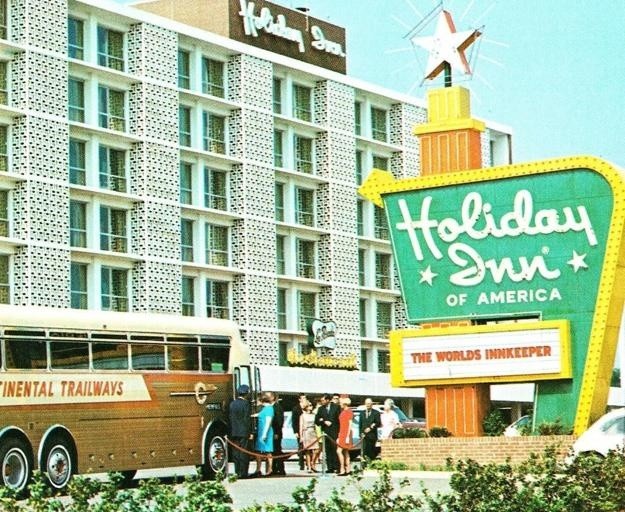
top-left (326, 469), bottom-right (335, 474)
top-left (300, 465), bottom-right (305, 470)
top-left (273, 470), bottom-right (286, 477)
top-left (238, 471), bottom-right (261, 479)
top-left (264, 471), bottom-right (273, 478)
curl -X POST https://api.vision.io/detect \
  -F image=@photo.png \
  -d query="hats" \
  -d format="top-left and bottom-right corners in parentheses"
top-left (237, 384), bottom-right (249, 397)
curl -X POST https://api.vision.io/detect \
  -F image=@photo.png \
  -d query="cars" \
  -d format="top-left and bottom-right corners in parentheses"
top-left (562, 407), bottom-right (625, 487)
top-left (280, 402), bottom-right (426, 462)
top-left (499, 415), bottom-right (533, 437)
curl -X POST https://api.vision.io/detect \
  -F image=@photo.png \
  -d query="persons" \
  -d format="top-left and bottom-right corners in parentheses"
top-left (228, 384), bottom-right (402, 479)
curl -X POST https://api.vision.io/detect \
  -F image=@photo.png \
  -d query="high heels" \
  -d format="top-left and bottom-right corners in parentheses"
top-left (337, 472), bottom-right (346, 477)
top-left (307, 468), bottom-right (312, 474)
top-left (344, 472), bottom-right (353, 477)
top-left (310, 468), bottom-right (319, 473)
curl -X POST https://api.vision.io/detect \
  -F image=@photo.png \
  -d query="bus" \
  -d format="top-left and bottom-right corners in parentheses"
top-left (0, 302), bottom-right (262, 501)
